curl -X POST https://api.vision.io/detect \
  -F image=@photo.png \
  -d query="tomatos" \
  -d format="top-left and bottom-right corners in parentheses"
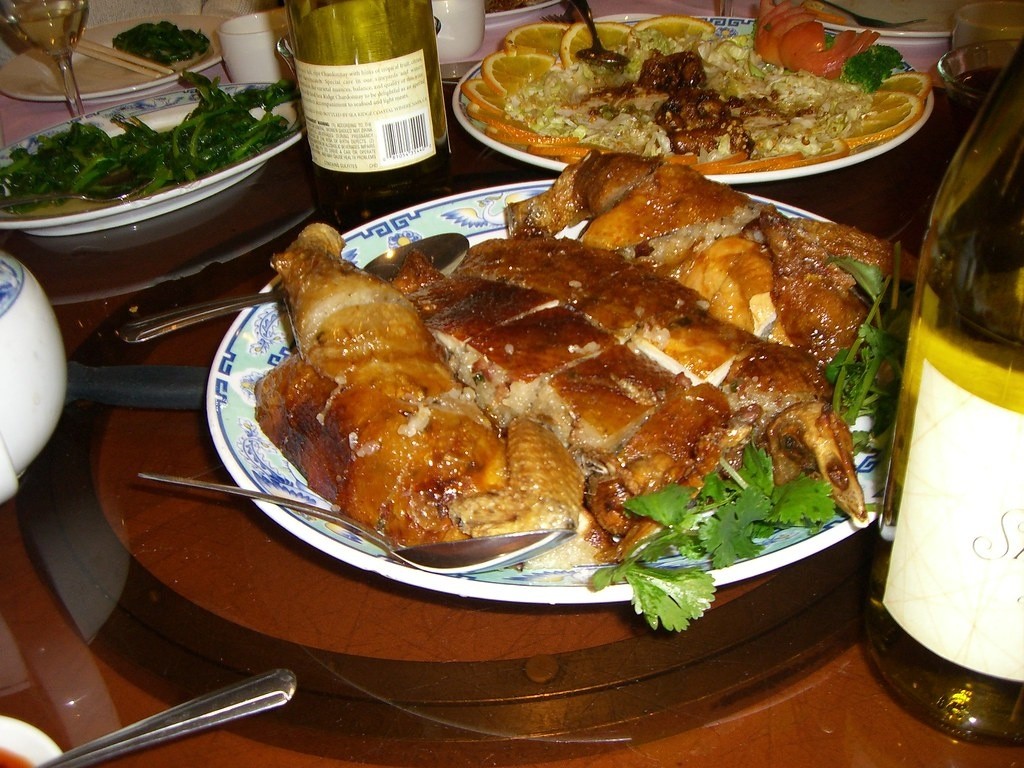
top-left (751, 0), bottom-right (881, 78)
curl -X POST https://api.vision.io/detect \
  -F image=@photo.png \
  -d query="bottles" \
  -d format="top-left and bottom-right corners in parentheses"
top-left (285, 0), bottom-right (452, 188)
top-left (861, 26), bottom-right (1024, 749)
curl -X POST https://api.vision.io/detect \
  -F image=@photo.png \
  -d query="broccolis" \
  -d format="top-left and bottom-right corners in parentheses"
top-left (823, 30), bottom-right (903, 92)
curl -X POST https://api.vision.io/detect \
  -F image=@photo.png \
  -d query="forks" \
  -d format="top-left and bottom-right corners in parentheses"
top-left (807, 0), bottom-right (928, 25)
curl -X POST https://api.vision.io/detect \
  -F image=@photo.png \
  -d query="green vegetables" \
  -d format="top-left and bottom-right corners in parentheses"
top-left (111, 20), bottom-right (211, 66)
top-left (0, 71), bottom-right (301, 216)
top-left (589, 239), bottom-right (914, 635)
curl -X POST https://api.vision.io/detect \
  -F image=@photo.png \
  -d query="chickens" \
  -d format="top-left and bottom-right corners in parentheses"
top-left (251, 145), bottom-right (907, 568)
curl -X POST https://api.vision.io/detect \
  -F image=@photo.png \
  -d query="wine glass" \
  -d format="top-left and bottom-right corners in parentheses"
top-left (0, 1), bottom-right (90, 118)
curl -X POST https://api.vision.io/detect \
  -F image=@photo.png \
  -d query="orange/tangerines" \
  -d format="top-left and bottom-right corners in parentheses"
top-left (724, 70), bottom-right (932, 173)
top-left (457, 15), bottom-right (749, 175)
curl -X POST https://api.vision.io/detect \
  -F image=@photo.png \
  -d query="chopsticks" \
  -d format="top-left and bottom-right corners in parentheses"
top-left (74, 38), bottom-right (174, 78)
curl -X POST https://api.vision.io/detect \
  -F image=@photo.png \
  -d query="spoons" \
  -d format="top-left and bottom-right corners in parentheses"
top-left (115, 230), bottom-right (469, 345)
top-left (569, 0), bottom-right (629, 66)
top-left (137, 470), bottom-right (577, 577)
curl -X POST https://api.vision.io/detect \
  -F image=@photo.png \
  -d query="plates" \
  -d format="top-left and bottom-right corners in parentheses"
top-left (203, 179), bottom-right (910, 602)
top-left (0, 14), bottom-right (221, 107)
top-left (0, 82), bottom-right (310, 237)
top-left (773, 0), bottom-right (954, 37)
top-left (451, 14), bottom-right (934, 185)
top-left (485, 0), bottom-right (562, 30)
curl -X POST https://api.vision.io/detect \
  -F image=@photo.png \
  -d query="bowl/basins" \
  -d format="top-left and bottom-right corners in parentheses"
top-left (0, 714), bottom-right (63, 768)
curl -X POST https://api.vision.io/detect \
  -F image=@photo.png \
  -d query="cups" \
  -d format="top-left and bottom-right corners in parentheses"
top-left (216, 8), bottom-right (288, 83)
top-left (952, 0), bottom-right (1024, 67)
top-left (432, 0), bottom-right (484, 60)
top-left (1, 248), bottom-right (70, 508)
top-left (937, 40), bottom-right (1018, 127)
